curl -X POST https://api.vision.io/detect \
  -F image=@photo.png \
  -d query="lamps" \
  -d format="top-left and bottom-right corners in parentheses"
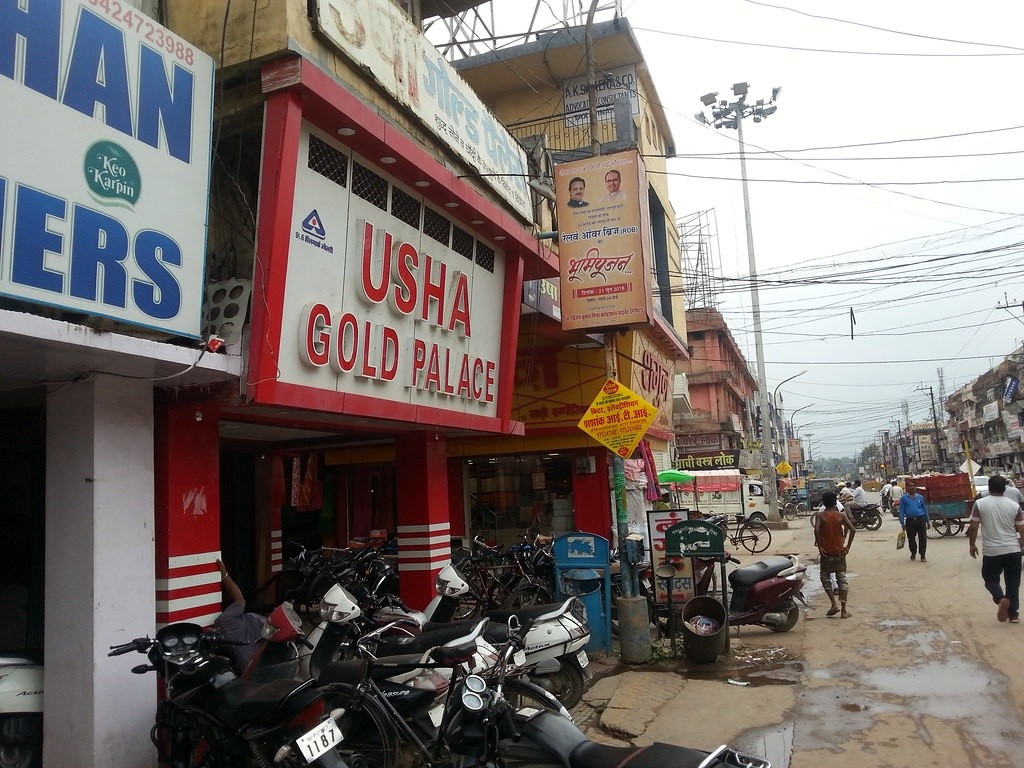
top-left (458, 173), bottom-right (556, 203)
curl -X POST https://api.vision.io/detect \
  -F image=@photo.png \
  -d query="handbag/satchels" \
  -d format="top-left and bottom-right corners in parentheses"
top-left (897, 531), bottom-right (905, 549)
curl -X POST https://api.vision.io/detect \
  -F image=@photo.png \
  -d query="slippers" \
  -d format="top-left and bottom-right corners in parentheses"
top-left (841, 613), bottom-right (852, 619)
top-left (826, 608), bottom-right (840, 615)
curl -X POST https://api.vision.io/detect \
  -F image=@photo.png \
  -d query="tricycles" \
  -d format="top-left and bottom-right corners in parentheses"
top-left (926, 500), bottom-right (981, 539)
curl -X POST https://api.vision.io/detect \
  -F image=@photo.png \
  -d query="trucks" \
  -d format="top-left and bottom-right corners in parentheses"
top-left (674, 470), bottom-right (771, 528)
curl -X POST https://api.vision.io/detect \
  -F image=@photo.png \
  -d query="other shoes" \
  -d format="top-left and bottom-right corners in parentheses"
top-left (1009, 610), bottom-right (1019, 622)
top-left (833, 588), bottom-right (839, 595)
top-left (997, 596), bottom-right (1010, 622)
top-left (921, 558), bottom-right (927, 562)
top-left (910, 553), bottom-right (915, 560)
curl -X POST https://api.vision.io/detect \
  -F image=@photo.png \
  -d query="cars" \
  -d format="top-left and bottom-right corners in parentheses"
top-left (974, 476), bottom-right (989, 497)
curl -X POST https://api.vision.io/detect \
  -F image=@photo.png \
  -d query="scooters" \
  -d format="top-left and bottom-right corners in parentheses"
top-left (488, 517), bottom-right (807, 638)
top-left (249, 547), bottom-right (591, 707)
top-left (882, 493), bottom-right (900, 517)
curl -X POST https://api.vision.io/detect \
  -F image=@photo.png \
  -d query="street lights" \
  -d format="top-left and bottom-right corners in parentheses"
top-left (791, 403), bottom-right (815, 478)
top-left (796, 422), bottom-right (821, 475)
top-left (774, 370), bottom-right (807, 462)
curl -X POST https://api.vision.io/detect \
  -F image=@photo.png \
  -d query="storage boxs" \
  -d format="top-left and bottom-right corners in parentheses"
top-left (672, 491), bottom-right (740, 504)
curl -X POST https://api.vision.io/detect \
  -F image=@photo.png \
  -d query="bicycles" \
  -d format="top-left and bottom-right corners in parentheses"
top-left (320, 616), bottom-right (581, 767)
top-left (785, 498), bottom-right (808, 520)
top-left (712, 513), bottom-right (770, 556)
top-left (286, 533), bottom-right (529, 611)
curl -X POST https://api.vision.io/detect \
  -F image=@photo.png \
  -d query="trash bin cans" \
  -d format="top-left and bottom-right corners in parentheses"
top-left (561, 568), bottom-right (604, 651)
top-left (681, 596), bottom-right (727, 661)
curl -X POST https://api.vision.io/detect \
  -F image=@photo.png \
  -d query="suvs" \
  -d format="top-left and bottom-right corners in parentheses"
top-left (808, 478), bottom-right (836, 510)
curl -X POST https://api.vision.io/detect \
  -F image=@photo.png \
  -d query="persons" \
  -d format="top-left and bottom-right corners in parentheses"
top-left (837, 479), bottom-right (867, 524)
top-left (749, 486), bottom-right (755, 496)
top-left (968, 475), bottom-right (1024, 623)
top-left (899, 481), bottom-right (931, 562)
top-left (882, 480), bottom-right (903, 514)
top-left (1000, 473), bottom-right (1024, 506)
top-left (567, 176), bottom-right (589, 208)
top-left (814, 492), bottom-right (856, 619)
top-left (599, 170), bottom-right (627, 202)
top-left (206, 560), bottom-right (270, 680)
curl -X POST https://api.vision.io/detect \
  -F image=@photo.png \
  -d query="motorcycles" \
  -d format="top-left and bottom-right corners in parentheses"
top-left (109, 623), bottom-right (349, 768)
top-left (442, 615), bottom-right (769, 767)
top-left (841, 499), bottom-right (882, 531)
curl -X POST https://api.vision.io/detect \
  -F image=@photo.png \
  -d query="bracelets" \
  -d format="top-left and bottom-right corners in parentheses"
top-left (222, 572), bottom-right (230, 580)
